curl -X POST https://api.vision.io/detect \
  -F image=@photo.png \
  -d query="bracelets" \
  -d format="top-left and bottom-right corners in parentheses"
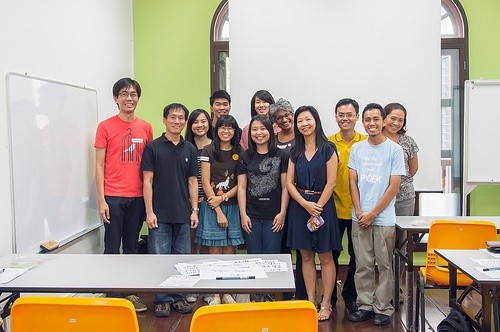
top-left (191, 208), bottom-right (199, 211)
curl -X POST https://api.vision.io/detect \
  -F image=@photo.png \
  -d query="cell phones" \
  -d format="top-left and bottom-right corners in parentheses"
top-left (307, 216), bottom-right (324, 233)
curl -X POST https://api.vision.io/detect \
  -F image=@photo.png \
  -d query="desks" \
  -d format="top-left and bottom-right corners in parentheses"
top-left (395, 216), bottom-right (500, 332)
top-left (434, 248), bottom-right (500, 332)
top-left (0, 254), bottom-right (297, 302)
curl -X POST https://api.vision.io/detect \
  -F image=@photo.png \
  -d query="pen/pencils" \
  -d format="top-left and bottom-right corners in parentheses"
top-left (216, 276), bottom-right (255, 280)
top-left (483, 267), bottom-right (500, 271)
top-left (0, 269), bottom-right (5, 274)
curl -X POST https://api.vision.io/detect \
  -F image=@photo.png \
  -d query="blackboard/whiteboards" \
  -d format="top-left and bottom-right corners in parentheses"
top-left (465, 80), bottom-right (500, 183)
top-left (6, 73), bottom-right (103, 254)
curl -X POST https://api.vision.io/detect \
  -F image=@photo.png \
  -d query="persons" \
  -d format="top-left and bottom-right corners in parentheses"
top-left (185, 108), bottom-right (215, 303)
top-left (94, 77), bottom-right (153, 312)
top-left (194, 114), bottom-right (245, 305)
top-left (286, 105), bottom-right (339, 321)
top-left (140, 103), bottom-right (199, 317)
top-left (383, 102), bottom-right (419, 303)
top-left (318, 98), bottom-right (369, 315)
top-left (346, 103), bottom-right (406, 325)
top-left (237, 113), bottom-right (289, 302)
top-left (270, 98), bottom-right (308, 301)
top-left (239, 90), bottom-right (275, 150)
top-left (206, 90), bottom-right (243, 143)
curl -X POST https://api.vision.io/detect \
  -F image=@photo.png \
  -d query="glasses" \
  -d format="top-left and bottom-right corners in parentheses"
top-left (118, 91), bottom-right (137, 97)
top-left (336, 112), bottom-right (357, 118)
top-left (218, 126), bottom-right (235, 131)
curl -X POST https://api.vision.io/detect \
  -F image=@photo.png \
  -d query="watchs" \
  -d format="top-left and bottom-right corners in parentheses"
top-left (222, 193), bottom-right (229, 202)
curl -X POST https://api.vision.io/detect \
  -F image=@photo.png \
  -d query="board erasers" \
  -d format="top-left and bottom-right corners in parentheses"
top-left (40, 240), bottom-right (59, 251)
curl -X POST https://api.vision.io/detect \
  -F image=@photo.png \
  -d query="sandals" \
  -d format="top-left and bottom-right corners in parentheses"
top-left (318, 304), bottom-right (333, 321)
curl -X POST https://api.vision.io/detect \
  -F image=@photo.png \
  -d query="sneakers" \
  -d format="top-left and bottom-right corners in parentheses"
top-left (125, 295), bottom-right (147, 311)
top-left (154, 303), bottom-right (171, 316)
top-left (171, 299), bottom-right (193, 313)
top-left (208, 296), bottom-right (221, 305)
top-left (222, 294), bottom-right (238, 304)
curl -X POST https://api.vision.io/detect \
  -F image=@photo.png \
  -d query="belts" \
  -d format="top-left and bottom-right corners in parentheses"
top-left (299, 189), bottom-right (322, 195)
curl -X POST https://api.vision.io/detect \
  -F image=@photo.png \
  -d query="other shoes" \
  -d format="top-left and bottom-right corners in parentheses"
top-left (186, 296), bottom-right (197, 302)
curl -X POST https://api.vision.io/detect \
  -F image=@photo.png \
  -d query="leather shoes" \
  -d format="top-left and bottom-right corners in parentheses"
top-left (371, 313), bottom-right (390, 326)
top-left (349, 309), bottom-right (376, 322)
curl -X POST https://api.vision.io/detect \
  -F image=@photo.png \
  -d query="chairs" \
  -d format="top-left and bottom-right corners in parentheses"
top-left (415, 220), bottom-right (498, 332)
top-left (10, 296), bottom-right (140, 332)
top-left (190, 300), bottom-right (319, 332)
